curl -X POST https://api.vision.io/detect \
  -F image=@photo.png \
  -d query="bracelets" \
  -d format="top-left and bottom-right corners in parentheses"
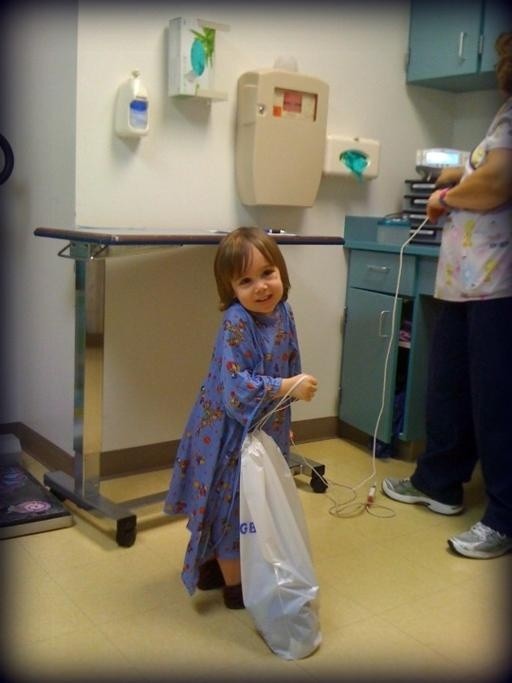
top-left (440, 189), bottom-right (454, 210)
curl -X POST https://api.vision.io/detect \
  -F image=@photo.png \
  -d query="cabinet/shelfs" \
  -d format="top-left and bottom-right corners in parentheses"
top-left (407, 0), bottom-right (512, 93)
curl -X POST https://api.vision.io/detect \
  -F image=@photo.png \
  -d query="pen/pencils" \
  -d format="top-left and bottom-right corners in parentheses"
top-left (263, 229), bottom-right (285, 233)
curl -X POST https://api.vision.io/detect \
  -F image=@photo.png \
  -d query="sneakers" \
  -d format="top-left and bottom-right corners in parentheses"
top-left (383, 478), bottom-right (464, 515)
top-left (448, 522), bottom-right (512, 558)
top-left (223, 586), bottom-right (243, 608)
top-left (199, 560), bottom-right (222, 590)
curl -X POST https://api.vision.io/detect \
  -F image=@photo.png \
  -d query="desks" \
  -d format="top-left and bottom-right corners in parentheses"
top-left (336, 236), bottom-right (441, 462)
top-left (33, 226), bottom-right (348, 549)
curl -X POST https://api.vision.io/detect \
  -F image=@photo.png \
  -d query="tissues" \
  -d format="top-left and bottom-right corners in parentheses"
top-left (170, 17), bottom-right (217, 103)
top-left (324, 134), bottom-right (381, 183)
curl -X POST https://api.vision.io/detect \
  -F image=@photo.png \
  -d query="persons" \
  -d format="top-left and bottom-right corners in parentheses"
top-left (163, 226), bottom-right (318, 612)
top-left (384, 25), bottom-right (510, 558)
